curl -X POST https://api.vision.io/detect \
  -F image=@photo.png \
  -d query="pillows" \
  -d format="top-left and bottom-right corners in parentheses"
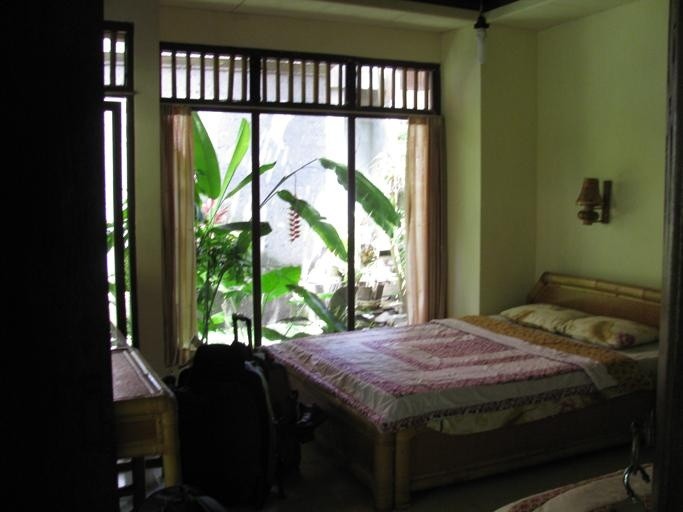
top-left (499, 302), bottom-right (587, 336)
top-left (556, 314), bottom-right (658, 349)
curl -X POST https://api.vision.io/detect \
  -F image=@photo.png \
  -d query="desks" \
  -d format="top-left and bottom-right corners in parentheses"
top-left (109, 349), bottom-right (180, 512)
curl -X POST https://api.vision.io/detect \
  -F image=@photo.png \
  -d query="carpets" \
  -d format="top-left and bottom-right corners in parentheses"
top-left (493, 462), bottom-right (661, 511)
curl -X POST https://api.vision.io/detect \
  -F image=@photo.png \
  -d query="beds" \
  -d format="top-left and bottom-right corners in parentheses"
top-left (252, 269), bottom-right (663, 512)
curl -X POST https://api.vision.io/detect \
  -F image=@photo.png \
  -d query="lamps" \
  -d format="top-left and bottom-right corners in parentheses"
top-left (573, 177), bottom-right (614, 227)
top-left (469, 6), bottom-right (492, 41)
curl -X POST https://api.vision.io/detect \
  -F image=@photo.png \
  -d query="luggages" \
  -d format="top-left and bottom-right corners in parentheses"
top-left (174, 313), bottom-right (303, 512)
